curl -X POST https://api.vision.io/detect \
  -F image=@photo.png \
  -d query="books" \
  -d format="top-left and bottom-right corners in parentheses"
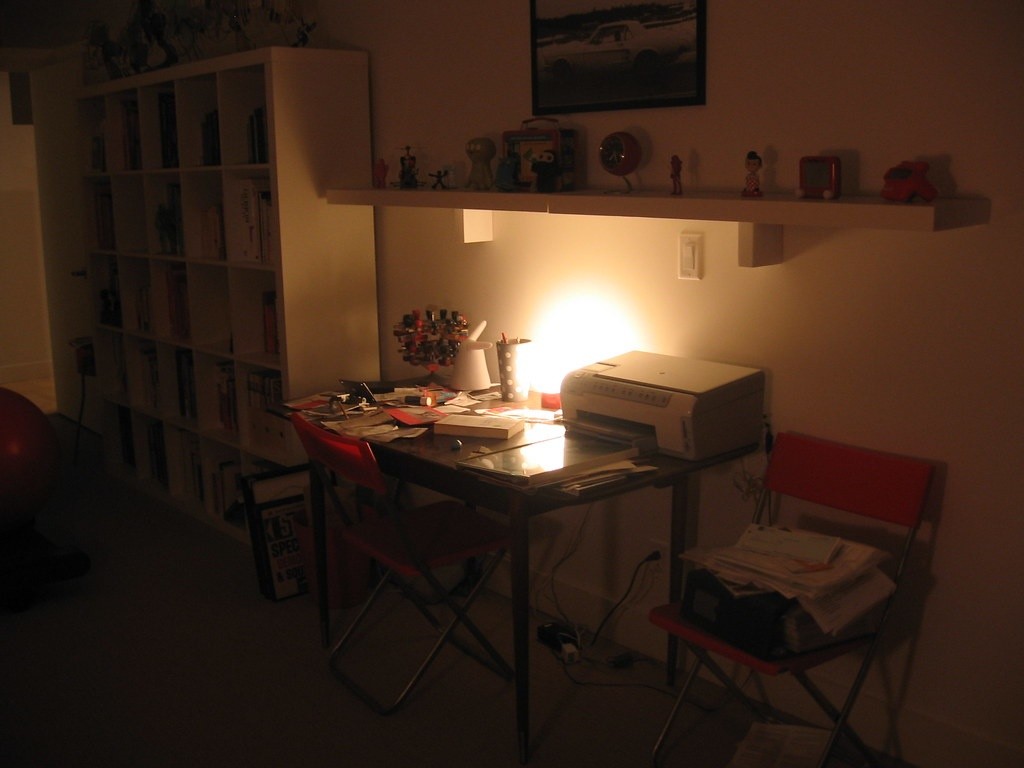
top-left (434, 413), bottom-right (526, 440)
top-left (454, 436), bottom-right (639, 486)
top-left (736, 523), bottom-right (843, 564)
top-left (100, 271), bottom-right (285, 529)
top-left (94, 184), bottom-right (274, 265)
top-left (90, 90), bottom-right (270, 174)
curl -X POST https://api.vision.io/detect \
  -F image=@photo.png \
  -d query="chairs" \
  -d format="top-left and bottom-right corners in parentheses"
top-left (648, 433), bottom-right (936, 768)
top-left (290, 413), bottom-right (515, 717)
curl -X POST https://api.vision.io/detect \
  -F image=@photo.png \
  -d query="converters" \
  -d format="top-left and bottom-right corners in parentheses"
top-left (537, 623), bottom-right (578, 652)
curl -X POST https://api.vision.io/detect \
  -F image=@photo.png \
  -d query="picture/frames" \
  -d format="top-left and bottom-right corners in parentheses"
top-left (529, 0), bottom-right (705, 116)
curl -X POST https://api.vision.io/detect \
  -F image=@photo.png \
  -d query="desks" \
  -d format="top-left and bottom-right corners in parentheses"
top-left (266, 374), bottom-right (759, 764)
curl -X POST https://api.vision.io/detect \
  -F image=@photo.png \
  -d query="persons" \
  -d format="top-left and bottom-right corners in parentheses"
top-left (744, 150), bottom-right (763, 192)
top-left (399, 146), bottom-right (416, 170)
top-left (669, 155), bottom-right (683, 196)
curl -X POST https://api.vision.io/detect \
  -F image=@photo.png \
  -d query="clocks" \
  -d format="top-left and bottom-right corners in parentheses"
top-left (598, 132), bottom-right (640, 193)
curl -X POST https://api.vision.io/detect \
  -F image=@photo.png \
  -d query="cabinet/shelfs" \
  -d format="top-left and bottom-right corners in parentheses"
top-left (76, 46), bottom-right (380, 549)
top-left (326, 186), bottom-right (988, 267)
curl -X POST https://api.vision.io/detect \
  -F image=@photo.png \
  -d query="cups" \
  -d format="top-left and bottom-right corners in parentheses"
top-left (496, 339), bottom-right (532, 402)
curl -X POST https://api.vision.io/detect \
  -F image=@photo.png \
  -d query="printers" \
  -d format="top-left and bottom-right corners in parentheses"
top-left (554, 350), bottom-right (768, 460)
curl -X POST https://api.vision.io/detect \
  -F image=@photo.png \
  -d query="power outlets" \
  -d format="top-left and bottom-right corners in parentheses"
top-left (649, 538), bottom-right (670, 570)
